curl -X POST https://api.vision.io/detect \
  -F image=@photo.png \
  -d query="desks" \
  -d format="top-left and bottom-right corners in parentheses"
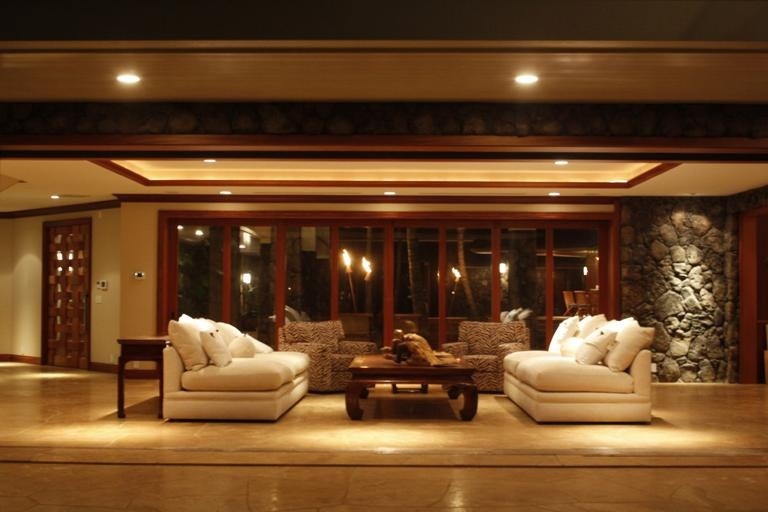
top-left (117, 339), bottom-right (165, 418)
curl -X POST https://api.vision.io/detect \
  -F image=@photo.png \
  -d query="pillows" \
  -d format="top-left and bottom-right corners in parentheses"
top-left (169, 314), bottom-right (273, 370)
top-left (548, 313), bottom-right (655, 372)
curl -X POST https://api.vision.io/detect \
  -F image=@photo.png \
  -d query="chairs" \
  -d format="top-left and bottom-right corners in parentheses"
top-left (337, 313), bottom-right (468, 343)
top-left (443, 320), bottom-right (532, 391)
top-left (277, 320), bottom-right (378, 393)
top-left (561, 284), bottom-right (600, 317)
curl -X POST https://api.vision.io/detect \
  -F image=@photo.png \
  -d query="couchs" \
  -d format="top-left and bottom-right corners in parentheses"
top-left (502, 349), bottom-right (652, 423)
top-left (161, 347), bottom-right (310, 421)
